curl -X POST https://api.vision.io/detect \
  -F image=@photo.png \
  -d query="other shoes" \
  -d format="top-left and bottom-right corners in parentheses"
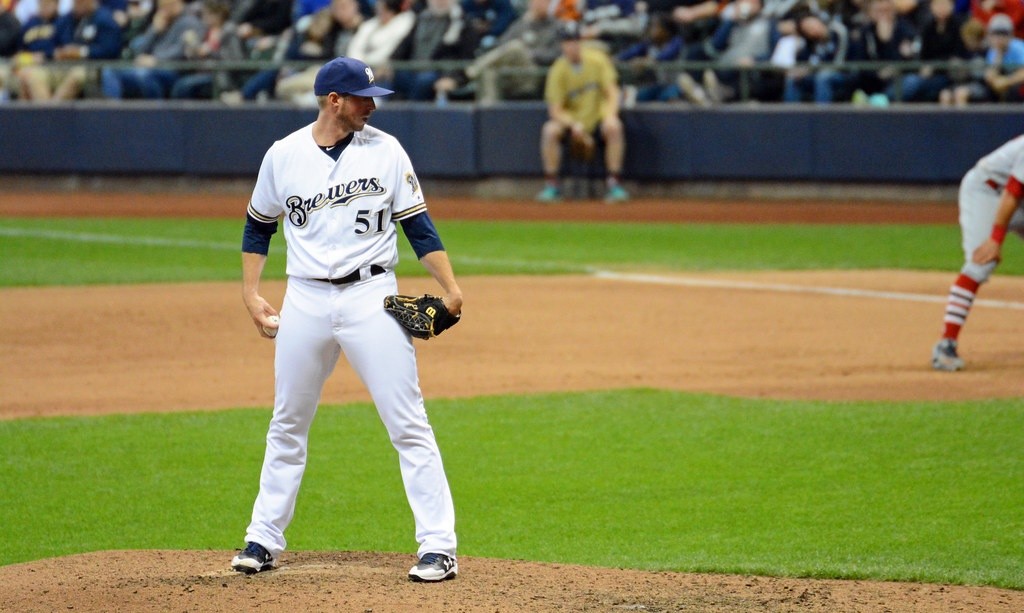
top-left (538, 185), bottom-right (560, 202)
top-left (603, 186), bottom-right (626, 201)
top-left (220, 89), bottom-right (243, 107)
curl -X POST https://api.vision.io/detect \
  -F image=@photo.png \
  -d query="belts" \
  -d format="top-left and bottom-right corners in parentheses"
top-left (985, 177), bottom-right (1000, 190)
top-left (315, 265), bottom-right (386, 284)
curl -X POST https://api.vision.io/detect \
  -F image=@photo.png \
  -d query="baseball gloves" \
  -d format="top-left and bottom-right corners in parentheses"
top-left (384, 295), bottom-right (462, 339)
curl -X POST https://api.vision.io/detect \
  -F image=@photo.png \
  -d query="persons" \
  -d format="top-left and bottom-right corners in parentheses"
top-left (535, 19), bottom-right (632, 204)
top-left (1, 1), bottom-right (1024, 105)
top-left (931, 132), bottom-right (1024, 373)
top-left (230, 56), bottom-right (465, 583)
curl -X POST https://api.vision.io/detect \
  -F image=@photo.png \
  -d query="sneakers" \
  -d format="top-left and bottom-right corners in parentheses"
top-left (932, 340), bottom-right (964, 372)
top-left (409, 551), bottom-right (458, 582)
top-left (230, 541), bottom-right (275, 572)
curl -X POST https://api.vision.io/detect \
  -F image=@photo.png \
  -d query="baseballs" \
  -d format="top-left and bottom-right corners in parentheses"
top-left (262, 316), bottom-right (281, 337)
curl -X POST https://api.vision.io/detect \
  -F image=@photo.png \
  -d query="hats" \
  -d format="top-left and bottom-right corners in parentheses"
top-left (987, 13), bottom-right (1014, 35)
top-left (960, 18), bottom-right (985, 44)
top-left (556, 20), bottom-right (580, 40)
top-left (314, 55), bottom-right (396, 97)
